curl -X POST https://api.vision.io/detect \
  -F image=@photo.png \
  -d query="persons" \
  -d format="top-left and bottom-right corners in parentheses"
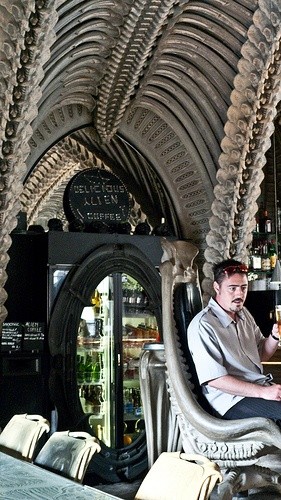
top-left (185, 259), bottom-right (281, 432)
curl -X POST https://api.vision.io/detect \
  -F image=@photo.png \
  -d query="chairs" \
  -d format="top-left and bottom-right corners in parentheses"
top-left (33, 432), bottom-right (101, 485)
top-left (0, 413), bottom-right (50, 463)
top-left (135, 452), bottom-right (223, 500)
top-left (160, 234), bottom-right (281, 500)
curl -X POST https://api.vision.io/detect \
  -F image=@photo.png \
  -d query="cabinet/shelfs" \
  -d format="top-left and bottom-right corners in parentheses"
top-left (243, 202), bottom-right (281, 338)
top-left (6, 231), bottom-right (168, 485)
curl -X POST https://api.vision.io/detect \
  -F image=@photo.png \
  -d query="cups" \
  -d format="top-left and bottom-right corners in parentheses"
top-left (275, 305), bottom-right (281, 349)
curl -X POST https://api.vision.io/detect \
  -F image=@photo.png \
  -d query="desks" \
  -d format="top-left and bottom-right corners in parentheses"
top-left (0, 452), bottom-right (124, 500)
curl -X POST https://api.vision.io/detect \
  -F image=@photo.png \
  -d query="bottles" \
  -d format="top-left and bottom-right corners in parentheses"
top-left (251, 200), bottom-right (281, 272)
top-left (76, 272), bottom-right (160, 450)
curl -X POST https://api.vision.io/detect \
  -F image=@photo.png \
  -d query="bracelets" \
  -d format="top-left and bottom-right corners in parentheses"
top-left (270, 329), bottom-right (280, 341)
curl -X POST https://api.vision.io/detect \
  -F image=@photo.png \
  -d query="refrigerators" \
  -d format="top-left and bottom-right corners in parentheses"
top-left (8, 233), bottom-right (176, 483)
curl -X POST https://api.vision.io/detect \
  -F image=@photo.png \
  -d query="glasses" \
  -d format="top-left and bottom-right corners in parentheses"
top-left (223, 265), bottom-right (248, 274)
